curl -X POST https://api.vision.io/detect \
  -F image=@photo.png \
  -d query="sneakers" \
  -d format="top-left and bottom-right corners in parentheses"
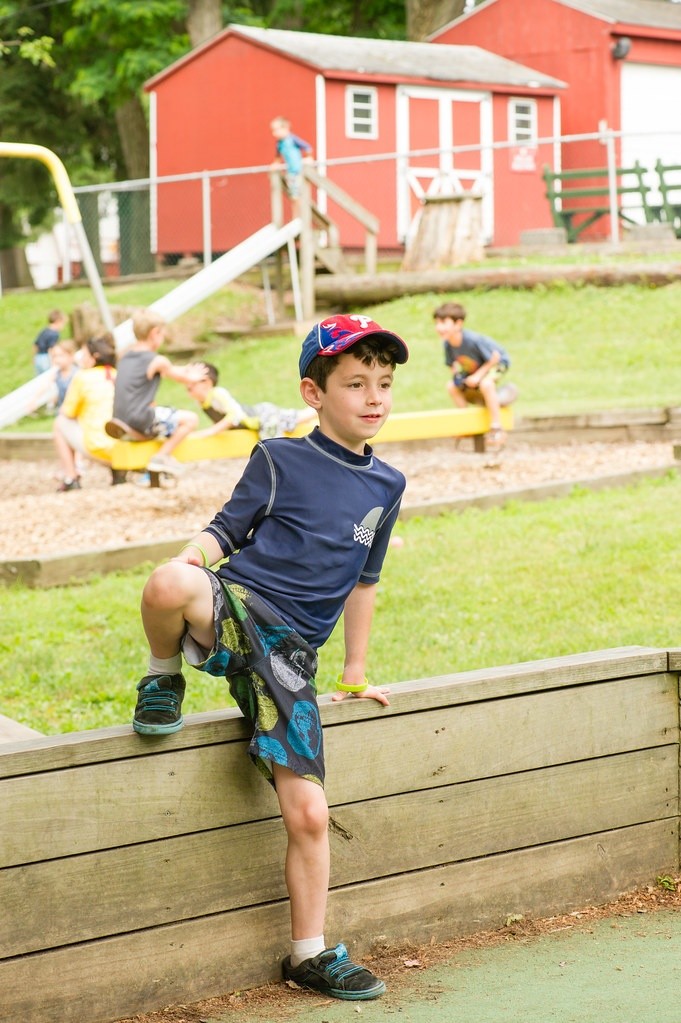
top-left (132, 670), bottom-right (186, 735)
top-left (280, 944), bottom-right (387, 1000)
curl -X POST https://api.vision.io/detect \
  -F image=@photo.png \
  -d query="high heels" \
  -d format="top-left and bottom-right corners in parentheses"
top-left (54, 475), bottom-right (82, 490)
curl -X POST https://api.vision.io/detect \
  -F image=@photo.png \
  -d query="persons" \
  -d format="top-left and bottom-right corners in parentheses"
top-left (33, 309), bottom-right (67, 374)
top-left (134, 316), bottom-right (409, 1000)
top-left (55, 332), bottom-right (117, 493)
top-left (188, 361), bottom-right (317, 441)
top-left (111, 315), bottom-right (209, 473)
top-left (50, 339), bottom-right (75, 408)
top-left (433, 301), bottom-right (510, 444)
top-left (270, 117), bottom-right (313, 219)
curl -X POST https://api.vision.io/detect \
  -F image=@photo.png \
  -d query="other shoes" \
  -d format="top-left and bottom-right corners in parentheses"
top-left (486, 427), bottom-right (506, 451)
top-left (147, 456), bottom-right (186, 472)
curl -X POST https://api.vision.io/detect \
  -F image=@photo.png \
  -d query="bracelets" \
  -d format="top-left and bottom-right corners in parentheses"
top-left (336, 672), bottom-right (368, 692)
top-left (178, 543), bottom-right (209, 569)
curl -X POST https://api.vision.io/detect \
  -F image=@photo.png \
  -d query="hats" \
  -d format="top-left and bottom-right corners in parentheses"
top-left (299, 314), bottom-right (409, 379)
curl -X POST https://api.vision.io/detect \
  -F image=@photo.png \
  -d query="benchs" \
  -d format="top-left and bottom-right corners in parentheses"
top-left (542, 159), bottom-right (681, 242)
